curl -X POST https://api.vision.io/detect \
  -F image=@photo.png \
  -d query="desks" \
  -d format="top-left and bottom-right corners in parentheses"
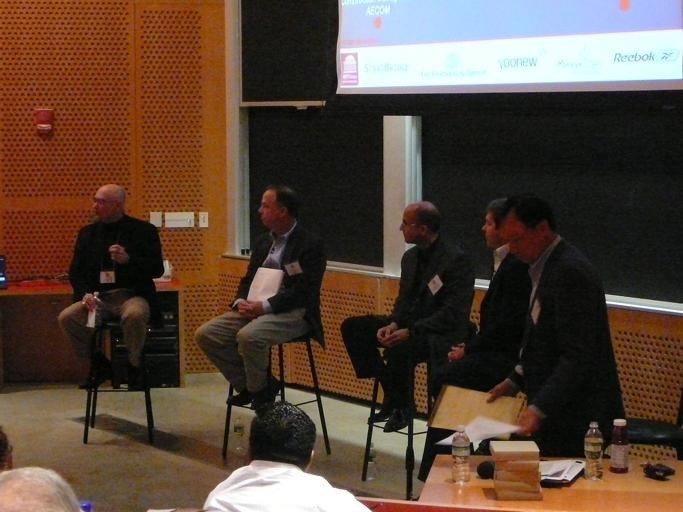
top-left (419, 456), bottom-right (683, 512)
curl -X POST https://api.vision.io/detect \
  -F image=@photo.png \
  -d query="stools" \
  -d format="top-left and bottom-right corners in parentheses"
top-left (221, 334), bottom-right (331, 460)
top-left (622, 420), bottom-right (682, 460)
top-left (361, 352), bottom-right (475, 501)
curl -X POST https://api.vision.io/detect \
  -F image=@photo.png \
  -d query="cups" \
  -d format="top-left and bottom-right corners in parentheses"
top-left (152, 259), bottom-right (173, 283)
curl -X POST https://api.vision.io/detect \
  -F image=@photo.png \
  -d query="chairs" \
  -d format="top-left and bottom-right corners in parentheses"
top-left (83, 318), bottom-right (156, 444)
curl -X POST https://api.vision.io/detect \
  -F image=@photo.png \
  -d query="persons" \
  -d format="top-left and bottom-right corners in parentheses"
top-left (201, 401), bottom-right (373, 512)
top-left (0, 422), bottom-right (83, 512)
top-left (339, 200), bottom-right (476, 432)
top-left (418, 196), bottom-right (532, 483)
top-left (193, 182), bottom-right (328, 411)
top-left (56, 182), bottom-right (165, 391)
top-left (485, 192), bottom-right (627, 457)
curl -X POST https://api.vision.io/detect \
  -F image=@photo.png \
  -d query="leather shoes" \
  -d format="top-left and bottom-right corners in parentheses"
top-left (78, 356), bottom-right (114, 390)
top-left (227, 387), bottom-right (251, 406)
top-left (368, 405), bottom-right (417, 432)
top-left (125, 357), bottom-right (149, 390)
top-left (251, 375), bottom-right (280, 410)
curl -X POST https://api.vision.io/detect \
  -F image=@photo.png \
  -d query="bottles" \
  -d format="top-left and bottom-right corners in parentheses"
top-left (233, 415), bottom-right (247, 457)
top-left (81, 502), bottom-right (91, 512)
top-left (609, 419), bottom-right (629, 473)
top-left (583, 419), bottom-right (605, 481)
top-left (86, 291), bottom-right (104, 328)
top-left (451, 424), bottom-right (471, 484)
top-left (364, 442), bottom-right (377, 480)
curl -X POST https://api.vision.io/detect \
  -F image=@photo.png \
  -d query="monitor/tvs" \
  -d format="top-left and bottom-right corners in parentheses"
top-left (0, 257), bottom-right (6, 289)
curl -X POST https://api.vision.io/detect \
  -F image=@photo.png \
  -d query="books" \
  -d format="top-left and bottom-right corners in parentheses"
top-left (246, 267), bottom-right (284, 303)
top-left (423, 383), bottom-right (529, 435)
